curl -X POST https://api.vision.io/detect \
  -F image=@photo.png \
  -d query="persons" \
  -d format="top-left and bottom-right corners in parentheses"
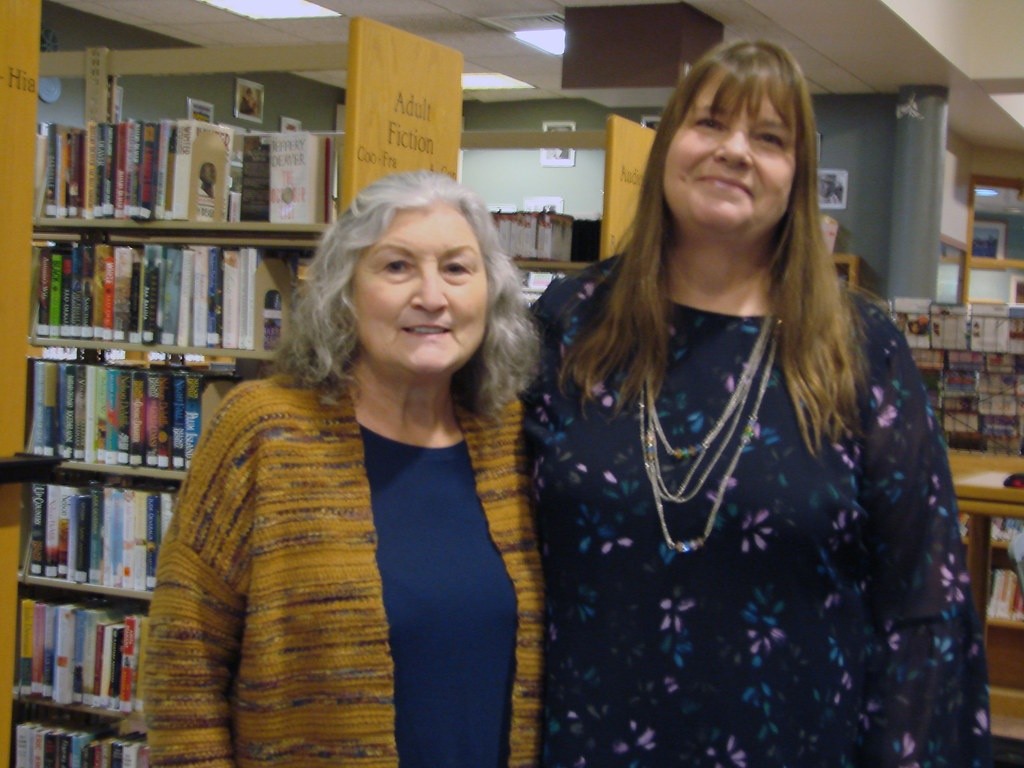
top-left (135, 171), bottom-right (546, 767)
top-left (524, 30), bottom-right (987, 767)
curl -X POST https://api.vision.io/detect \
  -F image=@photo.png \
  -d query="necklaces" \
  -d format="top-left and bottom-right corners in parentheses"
top-left (620, 276), bottom-right (784, 554)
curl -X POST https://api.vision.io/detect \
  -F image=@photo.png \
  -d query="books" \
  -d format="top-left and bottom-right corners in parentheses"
top-left (16, 112), bottom-right (340, 767)
top-left (885, 297), bottom-right (1023, 455)
top-left (483, 204), bottom-right (576, 261)
top-left (985, 512), bottom-right (1024, 625)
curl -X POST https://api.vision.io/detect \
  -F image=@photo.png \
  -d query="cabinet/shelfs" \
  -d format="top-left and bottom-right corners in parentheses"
top-left (469, 116), bottom-right (659, 333)
top-left (6, 20), bottom-right (463, 767)
top-left (932, 449), bottom-right (1024, 741)
top-left (874, 293), bottom-right (1024, 456)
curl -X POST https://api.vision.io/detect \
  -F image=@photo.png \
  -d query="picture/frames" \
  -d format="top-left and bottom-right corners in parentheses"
top-left (969, 217), bottom-right (1008, 265)
